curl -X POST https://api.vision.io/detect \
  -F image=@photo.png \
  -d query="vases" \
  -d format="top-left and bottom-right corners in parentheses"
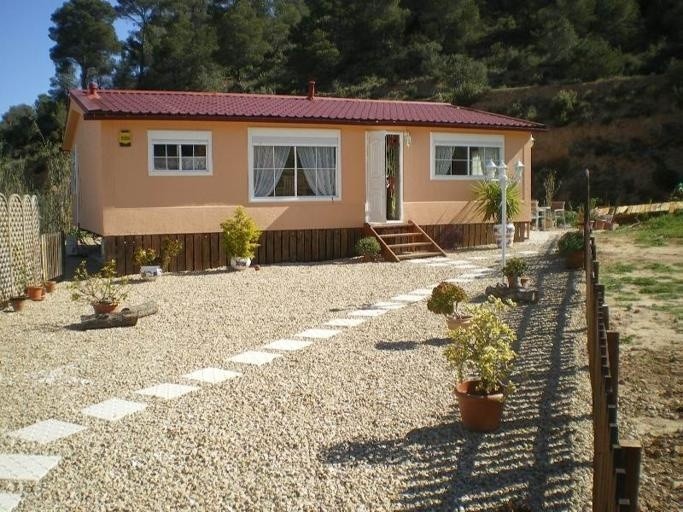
top-left (521, 278), bottom-right (531, 288)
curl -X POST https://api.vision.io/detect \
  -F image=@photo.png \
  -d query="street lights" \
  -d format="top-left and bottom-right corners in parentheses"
top-left (487, 159), bottom-right (526, 284)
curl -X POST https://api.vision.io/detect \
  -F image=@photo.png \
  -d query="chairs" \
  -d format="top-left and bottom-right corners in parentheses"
top-left (531, 200), bottom-right (565, 230)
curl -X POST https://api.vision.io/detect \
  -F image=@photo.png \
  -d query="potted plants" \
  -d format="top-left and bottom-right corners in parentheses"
top-left (426, 281), bottom-right (474, 334)
top-left (501, 255), bottom-right (528, 288)
top-left (558, 199), bottom-right (607, 268)
top-left (443, 295), bottom-right (537, 432)
top-left (220, 205), bottom-right (264, 270)
top-left (10, 238), bottom-right (183, 314)
top-left (469, 173), bottom-right (521, 248)
top-left (355, 237), bottom-right (382, 262)
